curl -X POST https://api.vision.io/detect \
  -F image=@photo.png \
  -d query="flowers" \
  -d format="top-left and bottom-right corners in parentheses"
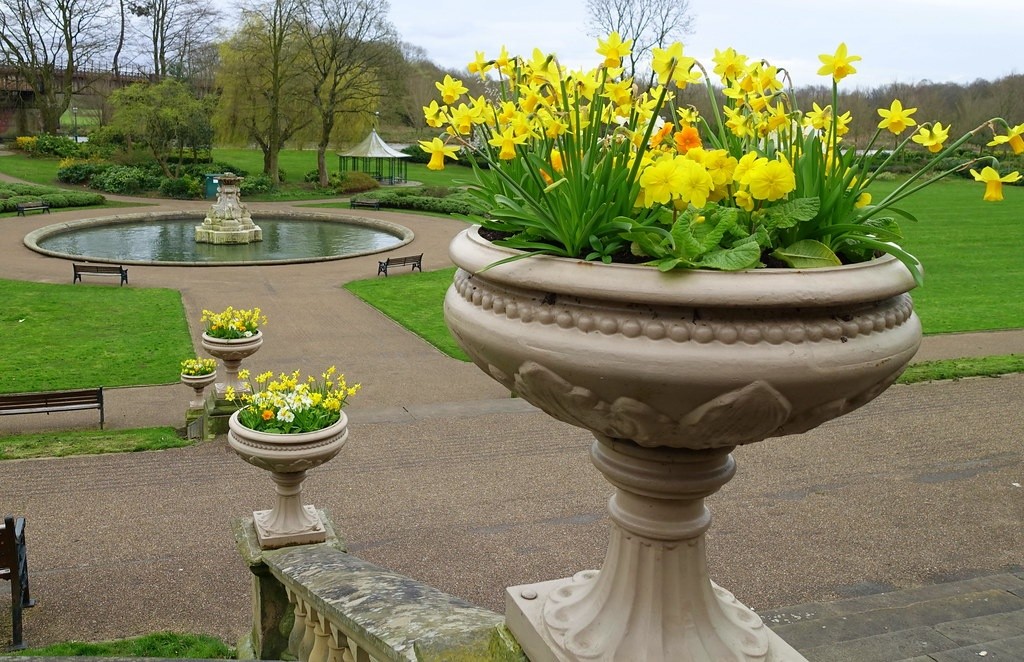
top-left (419, 29), bottom-right (1024, 273)
top-left (180, 357), bottom-right (216, 376)
top-left (200, 305), bottom-right (268, 339)
top-left (223, 364), bottom-right (363, 434)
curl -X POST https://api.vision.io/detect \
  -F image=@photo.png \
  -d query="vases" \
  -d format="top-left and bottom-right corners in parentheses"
top-left (443, 217), bottom-right (924, 662)
top-left (201, 330), bottom-right (263, 399)
top-left (226, 406), bottom-right (349, 551)
top-left (180, 371), bottom-right (217, 411)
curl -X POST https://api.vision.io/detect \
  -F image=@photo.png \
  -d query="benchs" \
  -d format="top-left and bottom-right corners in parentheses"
top-left (378, 253), bottom-right (423, 277)
top-left (350, 198), bottom-right (380, 211)
top-left (72, 257), bottom-right (128, 286)
top-left (17, 201), bottom-right (50, 216)
top-left (0, 386), bottom-right (104, 430)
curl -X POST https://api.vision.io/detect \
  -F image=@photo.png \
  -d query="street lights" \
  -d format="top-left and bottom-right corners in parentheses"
top-left (72, 106), bottom-right (78, 142)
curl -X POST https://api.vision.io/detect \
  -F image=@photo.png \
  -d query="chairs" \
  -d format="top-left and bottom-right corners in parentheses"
top-left (0, 515), bottom-right (37, 652)
